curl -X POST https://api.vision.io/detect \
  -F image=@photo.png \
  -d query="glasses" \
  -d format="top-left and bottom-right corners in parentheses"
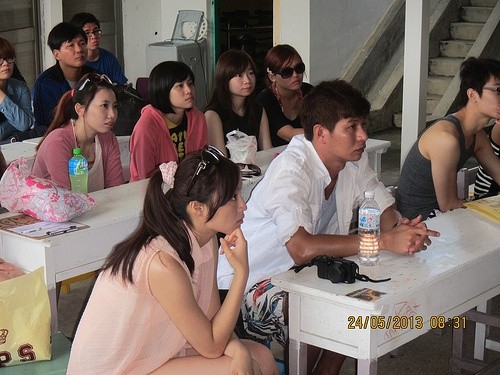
top-left (273, 63), bottom-right (306, 79)
top-left (85, 29), bottom-right (102, 37)
top-left (0, 56), bottom-right (16, 65)
top-left (78, 74), bottom-right (113, 91)
top-left (473, 87), bottom-right (500, 95)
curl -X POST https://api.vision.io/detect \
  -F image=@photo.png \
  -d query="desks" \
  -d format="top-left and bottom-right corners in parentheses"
top-left (0, 136), bottom-right (500, 375)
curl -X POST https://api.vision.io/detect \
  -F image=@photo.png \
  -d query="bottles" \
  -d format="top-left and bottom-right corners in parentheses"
top-left (68, 147), bottom-right (89, 194)
top-left (358, 191), bottom-right (381, 266)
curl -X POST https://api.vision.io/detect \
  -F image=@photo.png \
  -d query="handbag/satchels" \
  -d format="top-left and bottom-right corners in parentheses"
top-left (0, 265), bottom-right (52, 367)
top-left (0, 160), bottom-right (97, 222)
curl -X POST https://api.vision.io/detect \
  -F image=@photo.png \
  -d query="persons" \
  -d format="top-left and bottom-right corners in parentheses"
top-left (32, 73), bottom-right (125, 193)
top-left (71, 12), bottom-right (129, 85)
top-left (201, 49), bottom-right (274, 159)
top-left (215, 81), bottom-right (440, 375)
top-left (66, 150), bottom-right (280, 375)
top-left (128, 60), bottom-right (208, 183)
top-left (0, 36), bottom-right (34, 146)
top-left (32, 21), bottom-right (93, 137)
top-left (395, 57), bottom-right (500, 221)
top-left (0, 148), bottom-right (62, 309)
top-left (259, 44), bottom-right (315, 148)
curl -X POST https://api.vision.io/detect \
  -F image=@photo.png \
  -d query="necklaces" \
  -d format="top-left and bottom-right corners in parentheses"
top-left (275, 88), bottom-right (304, 113)
top-left (78, 142), bottom-right (92, 159)
